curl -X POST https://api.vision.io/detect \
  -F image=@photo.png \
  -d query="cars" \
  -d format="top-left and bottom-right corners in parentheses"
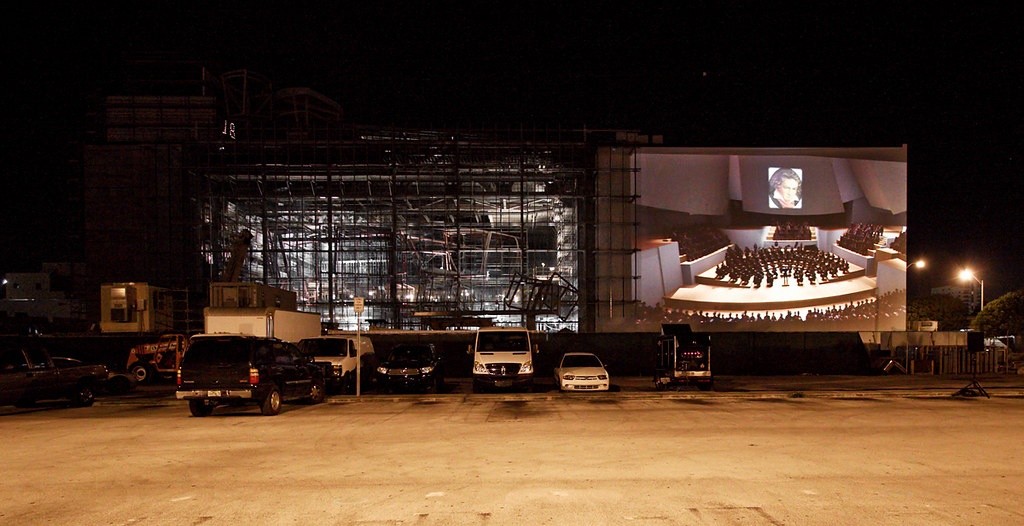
top-left (9, 354), bottom-right (140, 397)
top-left (373, 342), bottom-right (445, 393)
top-left (983, 335), bottom-right (1016, 354)
top-left (552, 352), bottom-right (610, 393)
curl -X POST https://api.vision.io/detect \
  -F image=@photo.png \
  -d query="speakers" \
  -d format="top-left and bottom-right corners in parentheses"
top-left (968, 332), bottom-right (984, 351)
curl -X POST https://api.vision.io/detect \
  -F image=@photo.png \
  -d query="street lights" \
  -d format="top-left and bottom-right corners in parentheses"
top-left (959, 269), bottom-right (983, 311)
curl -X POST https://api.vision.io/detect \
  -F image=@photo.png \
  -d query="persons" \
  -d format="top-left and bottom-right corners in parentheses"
top-left (632, 222), bottom-right (906, 324)
top-left (768, 168), bottom-right (803, 208)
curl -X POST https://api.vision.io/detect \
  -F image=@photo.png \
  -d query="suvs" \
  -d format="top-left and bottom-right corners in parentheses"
top-left (175, 332), bottom-right (326, 417)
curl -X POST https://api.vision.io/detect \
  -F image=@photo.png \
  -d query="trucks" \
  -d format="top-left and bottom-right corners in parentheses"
top-left (127, 333), bottom-right (187, 385)
top-left (653, 331), bottom-right (713, 390)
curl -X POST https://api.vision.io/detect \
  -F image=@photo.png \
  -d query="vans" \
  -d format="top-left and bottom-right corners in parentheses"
top-left (468, 326), bottom-right (538, 392)
top-left (294, 334), bottom-right (375, 394)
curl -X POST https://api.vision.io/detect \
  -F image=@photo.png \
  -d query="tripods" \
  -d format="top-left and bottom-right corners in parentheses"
top-left (952, 351), bottom-right (990, 399)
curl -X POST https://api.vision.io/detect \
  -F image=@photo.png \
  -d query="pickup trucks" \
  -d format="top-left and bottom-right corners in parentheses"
top-left (0, 342), bottom-right (110, 408)
